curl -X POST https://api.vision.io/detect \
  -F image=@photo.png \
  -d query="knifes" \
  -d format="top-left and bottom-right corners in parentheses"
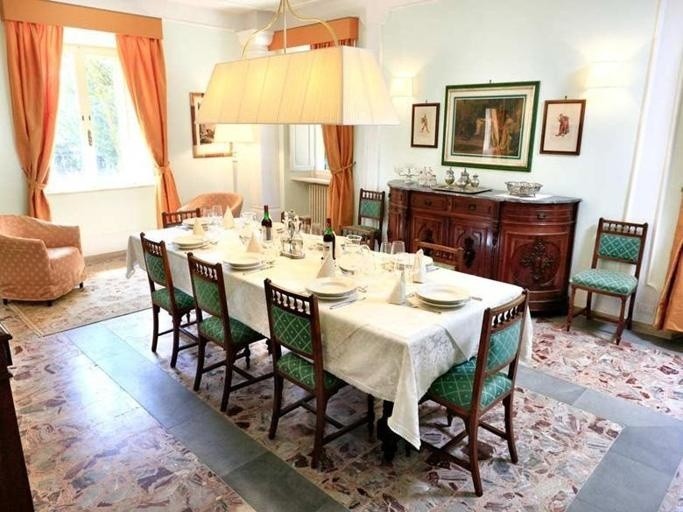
top-left (329, 297), bottom-right (357, 309)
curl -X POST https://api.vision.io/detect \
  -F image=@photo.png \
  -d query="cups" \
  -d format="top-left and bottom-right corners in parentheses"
top-left (339, 233), bottom-right (407, 288)
top-left (260, 240), bottom-right (276, 267)
top-left (203, 206), bottom-right (257, 246)
top-left (299, 222), bottom-right (321, 249)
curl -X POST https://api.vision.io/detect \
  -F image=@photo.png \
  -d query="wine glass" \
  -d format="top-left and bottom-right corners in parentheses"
top-left (398, 168), bottom-right (421, 184)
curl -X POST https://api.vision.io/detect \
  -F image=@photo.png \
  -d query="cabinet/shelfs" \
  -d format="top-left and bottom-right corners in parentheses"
top-left (503, 200), bottom-right (575, 320)
top-left (407, 189), bottom-right (501, 280)
top-left (386, 184), bottom-right (408, 250)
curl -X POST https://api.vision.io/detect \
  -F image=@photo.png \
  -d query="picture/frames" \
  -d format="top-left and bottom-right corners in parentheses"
top-left (538, 99), bottom-right (586, 157)
top-left (410, 101), bottom-right (440, 149)
top-left (439, 79), bottom-right (542, 174)
top-left (187, 90), bottom-right (234, 161)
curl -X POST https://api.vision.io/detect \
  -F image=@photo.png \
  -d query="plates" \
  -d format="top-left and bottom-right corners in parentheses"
top-left (174, 235), bottom-right (208, 250)
top-left (392, 252), bottom-right (433, 267)
top-left (305, 277), bottom-right (357, 299)
top-left (183, 217), bottom-right (210, 228)
top-left (222, 253), bottom-right (263, 270)
top-left (416, 286), bottom-right (470, 308)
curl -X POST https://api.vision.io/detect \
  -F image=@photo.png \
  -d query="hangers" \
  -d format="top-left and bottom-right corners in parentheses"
top-left (7, 256), bottom-right (166, 338)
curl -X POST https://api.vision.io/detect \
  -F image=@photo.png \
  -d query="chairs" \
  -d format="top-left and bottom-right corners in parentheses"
top-left (0, 211), bottom-right (85, 310)
top-left (177, 192), bottom-right (247, 224)
top-left (572, 214), bottom-right (650, 347)
top-left (342, 186), bottom-right (387, 249)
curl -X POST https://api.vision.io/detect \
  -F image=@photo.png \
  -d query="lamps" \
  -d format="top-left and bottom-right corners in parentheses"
top-left (194, 4), bottom-right (404, 132)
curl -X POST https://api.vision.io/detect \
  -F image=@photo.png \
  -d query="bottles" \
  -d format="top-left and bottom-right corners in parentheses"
top-left (321, 217), bottom-right (335, 261)
top-left (260, 205), bottom-right (272, 242)
top-left (278, 220), bottom-right (304, 256)
top-left (417, 170), bottom-right (437, 188)
top-left (444, 167), bottom-right (480, 191)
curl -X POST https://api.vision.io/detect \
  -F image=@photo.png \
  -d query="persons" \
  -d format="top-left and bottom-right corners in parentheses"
top-left (420, 114), bottom-right (429, 133)
top-left (499, 112), bottom-right (513, 150)
top-left (472, 117), bottom-right (482, 135)
top-left (554, 113), bottom-right (569, 137)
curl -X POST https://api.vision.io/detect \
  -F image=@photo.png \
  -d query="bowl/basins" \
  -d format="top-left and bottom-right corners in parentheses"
top-left (505, 181), bottom-right (542, 198)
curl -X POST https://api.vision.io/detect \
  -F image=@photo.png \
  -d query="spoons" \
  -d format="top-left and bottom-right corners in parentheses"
top-left (404, 298), bottom-right (442, 313)
